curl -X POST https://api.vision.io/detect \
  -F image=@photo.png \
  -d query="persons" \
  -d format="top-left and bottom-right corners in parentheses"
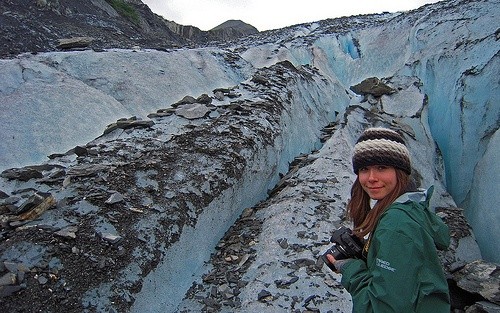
top-left (327, 126), bottom-right (452, 312)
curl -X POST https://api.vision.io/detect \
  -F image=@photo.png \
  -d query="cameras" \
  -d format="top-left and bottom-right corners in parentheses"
top-left (321, 227), bottom-right (368, 272)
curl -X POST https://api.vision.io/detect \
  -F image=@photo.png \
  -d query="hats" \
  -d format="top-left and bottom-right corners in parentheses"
top-left (352, 127), bottom-right (410, 174)
